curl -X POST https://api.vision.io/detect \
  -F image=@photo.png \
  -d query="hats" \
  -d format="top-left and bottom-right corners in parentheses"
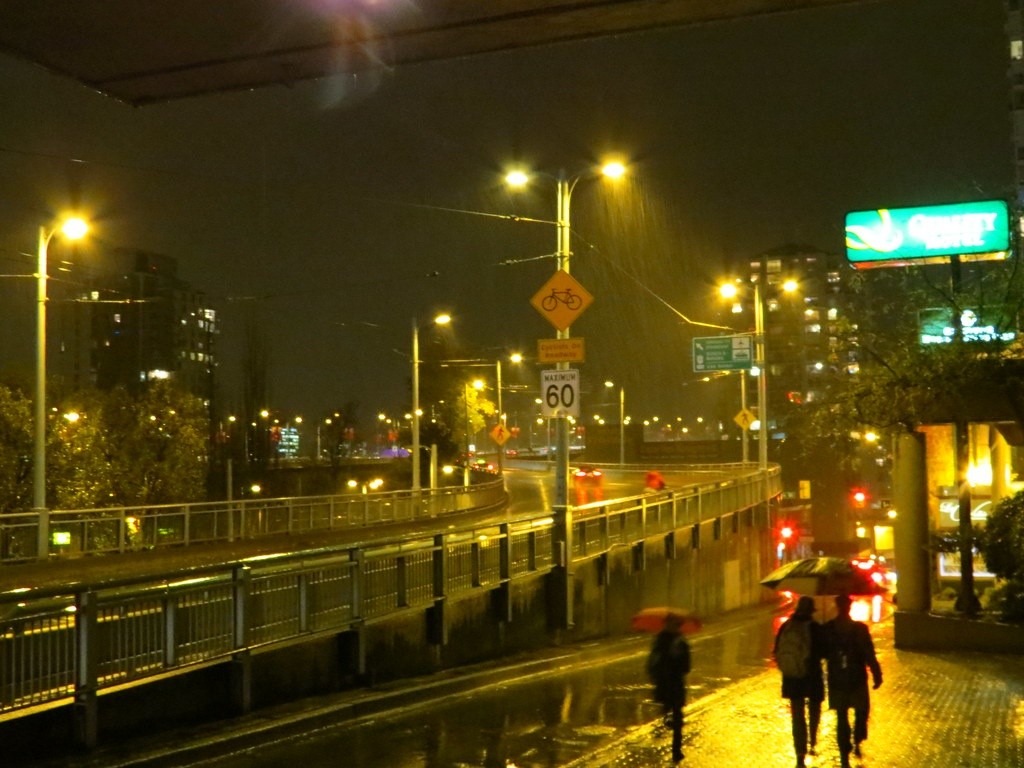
top-left (836, 596), bottom-right (851, 604)
top-left (797, 596), bottom-right (816, 612)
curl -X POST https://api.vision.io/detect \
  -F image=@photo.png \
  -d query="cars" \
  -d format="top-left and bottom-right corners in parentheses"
top-left (571, 464), bottom-right (603, 484)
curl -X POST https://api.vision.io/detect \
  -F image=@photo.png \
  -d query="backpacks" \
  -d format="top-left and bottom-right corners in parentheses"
top-left (824, 636), bottom-right (864, 688)
top-left (776, 620), bottom-right (817, 679)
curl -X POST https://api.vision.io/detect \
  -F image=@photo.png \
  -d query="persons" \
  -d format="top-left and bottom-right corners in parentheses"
top-left (647, 615), bottom-right (692, 762)
top-left (822, 594), bottom-right (882, 768)
top-left (774, 596), bottom-right (828, 768)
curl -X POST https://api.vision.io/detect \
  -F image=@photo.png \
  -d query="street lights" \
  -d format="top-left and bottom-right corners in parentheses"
top-left (30, 211), bottom-right (92, 563)
top-left (463, 379), bottom-right (484, 491)
top-left (721, 275), bottom-right (798, 470)
top-left (503, 157), bottom-right (627, 563)
top-left (412, 313), bottom-right (451, 516)
top-left (497, 353), bottom-right (524, 476)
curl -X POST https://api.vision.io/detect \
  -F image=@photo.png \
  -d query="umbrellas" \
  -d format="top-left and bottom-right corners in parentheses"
top-left (632, 608), bottom-right (702, 634)
top-left (758, 556), bottom-right (887, 623)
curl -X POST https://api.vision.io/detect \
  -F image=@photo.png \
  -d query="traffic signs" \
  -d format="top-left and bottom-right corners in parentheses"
top-left (691, 331), bottom-right (753, 373)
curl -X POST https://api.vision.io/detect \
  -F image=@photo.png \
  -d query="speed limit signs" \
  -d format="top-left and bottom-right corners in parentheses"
top-left (539, 369), bottom-right (579, 423)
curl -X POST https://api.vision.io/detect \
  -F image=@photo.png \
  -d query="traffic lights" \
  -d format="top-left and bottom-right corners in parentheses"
top-left (850, 487), bottom-right (870, 509)
top-left (777, 521), bottom-right (797, 547)
